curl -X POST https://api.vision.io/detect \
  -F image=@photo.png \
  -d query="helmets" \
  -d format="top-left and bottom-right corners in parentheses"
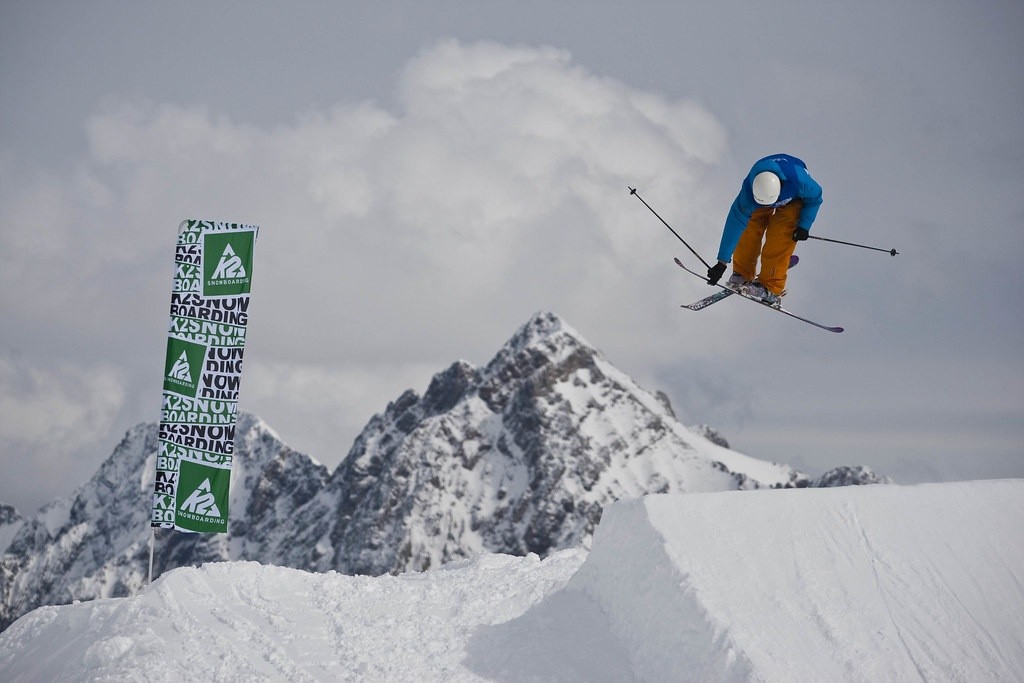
top-left (753, 171), bottom-right (781, 205)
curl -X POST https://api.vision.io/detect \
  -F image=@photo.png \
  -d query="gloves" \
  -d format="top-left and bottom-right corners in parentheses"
top-left (707, 261), bottom-right (727, 286)
top-left (792, 227), bottom-right (809, 242)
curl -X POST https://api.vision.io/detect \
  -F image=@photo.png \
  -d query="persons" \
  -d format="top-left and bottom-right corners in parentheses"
top-left (707, 153), bottom-right (824, 307)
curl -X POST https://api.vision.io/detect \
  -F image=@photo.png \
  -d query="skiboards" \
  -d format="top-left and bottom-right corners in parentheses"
top-left (674, 255), bottom-right (844, 334)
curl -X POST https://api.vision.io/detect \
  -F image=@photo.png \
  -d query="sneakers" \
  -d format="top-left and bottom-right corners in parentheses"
top-left (729, 271), bottom-right (758, 289)
top-left (741, 282), bottom-right (781, 309)
